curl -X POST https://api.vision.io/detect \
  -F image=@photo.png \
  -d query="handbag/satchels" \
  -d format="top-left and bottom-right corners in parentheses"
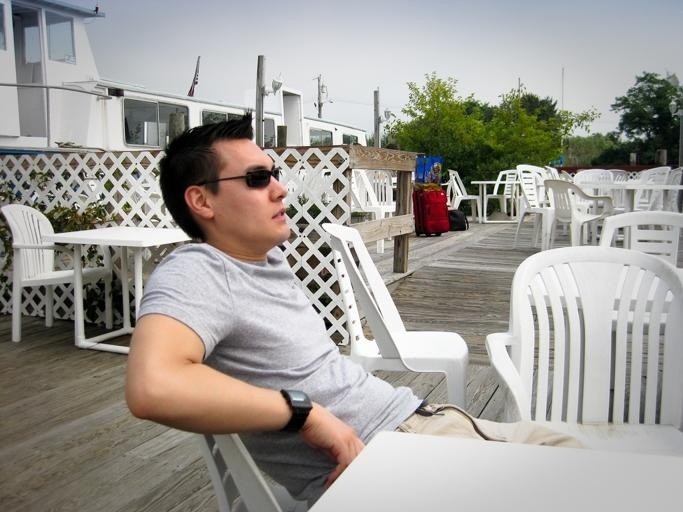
top-left (416, 155), bottom-right (443, 184)
top-left (449, 209), bottom-right (469, 230)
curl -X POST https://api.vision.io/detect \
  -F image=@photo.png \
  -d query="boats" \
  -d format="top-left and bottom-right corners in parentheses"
top-left (0, 0), bottom-right (367, 207)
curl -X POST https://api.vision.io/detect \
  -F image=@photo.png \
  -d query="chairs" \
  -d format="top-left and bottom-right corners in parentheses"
top-left (484, 246), bottom-right (682, 429)
top-left (351, 163), bottom-right (414, 256)
top-left (440, 165), bottom-right (682, 269)
top-left (320, 221), bottom-right (469, 411)
top-left (199, 425), bottom-right (304, 512)
top-left (0, 203), bottom-right (112, 343)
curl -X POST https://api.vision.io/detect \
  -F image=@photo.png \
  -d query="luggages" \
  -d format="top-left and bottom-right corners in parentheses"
top-left (412, 190), bottom-right (450, 237)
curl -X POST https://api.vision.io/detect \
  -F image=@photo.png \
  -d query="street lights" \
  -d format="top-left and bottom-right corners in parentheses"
top-left (255, 54), bottom-right (281, 149)
top-left (668, 98), bottom-right (682, 211)
top-left (371, 90), bottom-right (390, 147)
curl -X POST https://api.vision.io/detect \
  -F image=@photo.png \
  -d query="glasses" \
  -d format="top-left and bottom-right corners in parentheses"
top-left (200, 167), bottom-right (280, 187)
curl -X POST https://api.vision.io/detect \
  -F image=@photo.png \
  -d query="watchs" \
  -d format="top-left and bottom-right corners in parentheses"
top-left (280, 389), bottom-right (313, 433)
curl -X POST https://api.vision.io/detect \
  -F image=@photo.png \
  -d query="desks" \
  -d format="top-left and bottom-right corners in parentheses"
top-left (306, 430), bottom-right (683, 510)
top-left (40, 226), bottom-right (195, 356)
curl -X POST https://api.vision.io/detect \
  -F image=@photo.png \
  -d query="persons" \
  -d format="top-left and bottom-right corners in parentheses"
top-left (124, 111), bottom-right (600, 510)
